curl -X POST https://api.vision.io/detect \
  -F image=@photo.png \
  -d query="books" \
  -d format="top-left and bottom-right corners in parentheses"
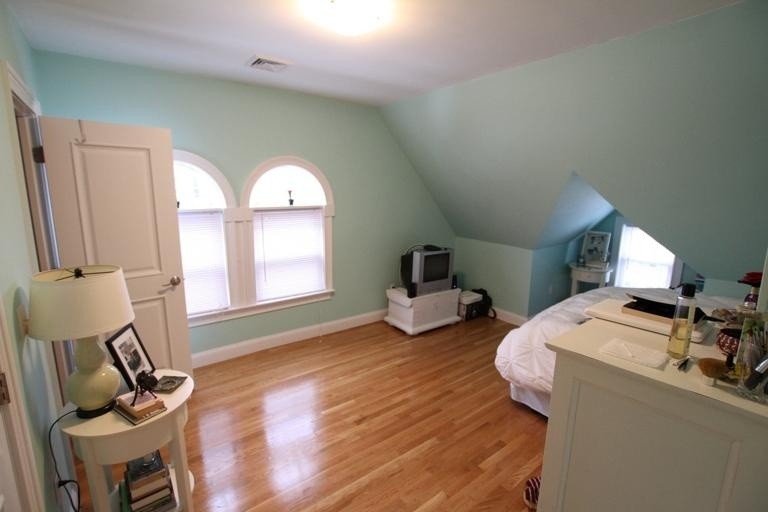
top-left (153, 375), bottom-right (188, 394)
top-left (116, 389), bottom-right (164, 416)
top-left (119, 448), bottom-right (177, 511)
top-left (113, 405), bottom-right (167, 426)
top-left (619, 292), bottom-right (708, 330)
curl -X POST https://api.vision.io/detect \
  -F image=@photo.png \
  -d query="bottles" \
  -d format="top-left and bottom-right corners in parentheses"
top-left (667, 283), bottom-right (698, 360)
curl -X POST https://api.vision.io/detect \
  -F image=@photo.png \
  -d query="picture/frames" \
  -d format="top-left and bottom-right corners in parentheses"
top-left (581, 231), bottom-right (612, 269)
top-left (105, 323), bottom-right (155, 391)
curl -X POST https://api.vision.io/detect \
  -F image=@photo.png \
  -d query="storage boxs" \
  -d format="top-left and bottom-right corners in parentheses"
top-left (458, 290), bottom-right (483, 322)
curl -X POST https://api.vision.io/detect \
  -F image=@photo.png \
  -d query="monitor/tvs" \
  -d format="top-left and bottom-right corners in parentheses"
top-left (401, 248), bottom-right (454, 297)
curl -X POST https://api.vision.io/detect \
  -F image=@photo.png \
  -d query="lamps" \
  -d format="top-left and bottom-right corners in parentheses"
top-left (29, 264), bottom-right (135, 419)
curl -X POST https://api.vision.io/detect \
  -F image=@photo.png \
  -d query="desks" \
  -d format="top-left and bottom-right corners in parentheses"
top-left (569, 263), bottom-right (613, 297)
top-left (56, 368), bottom-right (194, 512)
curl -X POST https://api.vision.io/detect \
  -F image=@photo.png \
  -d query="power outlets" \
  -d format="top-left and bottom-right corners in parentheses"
top-left (16, 303), bottom-right (30, 337)
top-left (54, 474), bottom-right (62, 502)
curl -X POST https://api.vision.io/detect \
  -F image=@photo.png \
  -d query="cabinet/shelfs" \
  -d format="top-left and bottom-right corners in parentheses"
top-left (535, 316), bottom-right (768, 512)
top-left (384, 287), bottom-right (463, 336)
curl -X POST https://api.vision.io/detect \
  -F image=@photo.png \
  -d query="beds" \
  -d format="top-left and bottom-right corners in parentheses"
top-left (496, 285), bottom-right (744, 420)
